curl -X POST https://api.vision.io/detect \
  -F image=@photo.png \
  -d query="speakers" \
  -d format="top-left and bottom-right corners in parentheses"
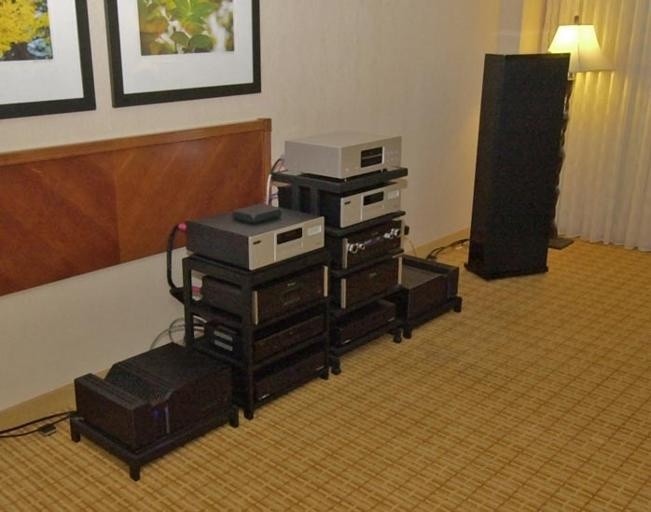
top-left (465, 52), bottom-right (571, 282)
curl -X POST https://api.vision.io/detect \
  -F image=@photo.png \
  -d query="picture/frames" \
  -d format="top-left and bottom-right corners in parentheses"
top-left (0, 1), bottom-right (262, 119)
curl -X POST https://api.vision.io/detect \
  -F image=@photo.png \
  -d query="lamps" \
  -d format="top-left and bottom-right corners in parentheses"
top-left (548, 15), bottom-right (617, 248)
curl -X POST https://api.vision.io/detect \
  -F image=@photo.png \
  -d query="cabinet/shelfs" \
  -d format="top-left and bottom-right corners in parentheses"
top-left (180, 205), bottom-right (331, 422)
top-left (271, 166), bottom-right (410, 377)
